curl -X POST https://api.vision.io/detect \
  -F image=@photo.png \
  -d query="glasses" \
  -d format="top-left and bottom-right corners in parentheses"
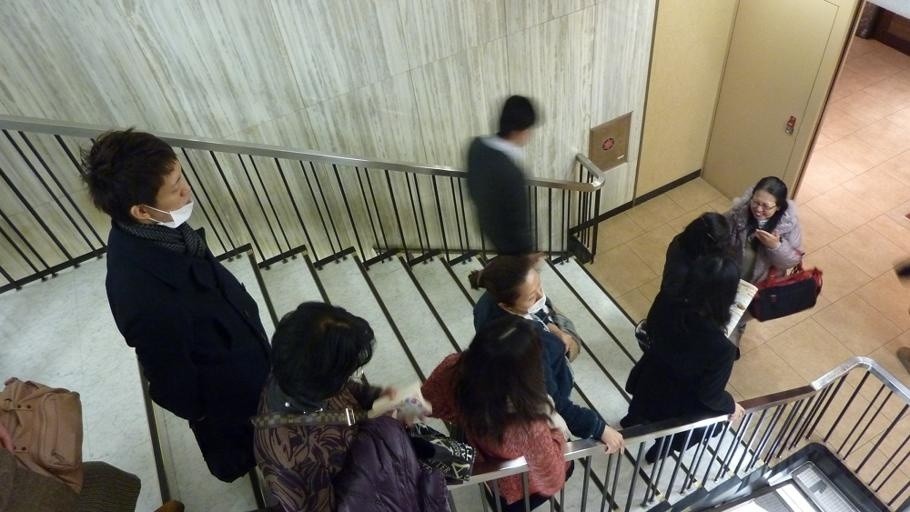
top-left (749, 197), bottom-right (777, 211)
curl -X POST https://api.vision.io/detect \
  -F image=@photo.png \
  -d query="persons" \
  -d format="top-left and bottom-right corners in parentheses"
top-left (78, 127), bottom-right (272, 483)
top-left (467, 253), bottom-right (624, 455)
top-left (623, 253), bottom-right (746, 463)
top-left (422, 314), bottom-right (574, 512)
top-left (0, 447), bottom-right (185, 512)
top-left (722, 177), bottom-right (801, 360)
top-left (465, 96), bottom-right (537, 253)
top-left (252, 302), bottom-right (451, 512)
top-left (635, 212), bottom-right (729, 353)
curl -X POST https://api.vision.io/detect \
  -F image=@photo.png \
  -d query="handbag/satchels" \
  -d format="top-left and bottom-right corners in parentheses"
top-left (1, 378), bottom-right (84, 492)
top-left (748, 249), bottom-right (823, 322)
top-left (407, 420), bottom-right (476, 484)
top-left (553, 313), bottom-right (580, 363)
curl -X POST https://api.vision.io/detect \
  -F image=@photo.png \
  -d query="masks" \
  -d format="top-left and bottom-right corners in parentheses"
top-left (527, 293), bottom-right (548, 317)
top-left (142, 198), bottom-right (195, 232)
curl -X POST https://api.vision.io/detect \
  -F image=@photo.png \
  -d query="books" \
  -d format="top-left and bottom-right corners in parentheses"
top-left (367, 382), bottom-right (433, 423)
